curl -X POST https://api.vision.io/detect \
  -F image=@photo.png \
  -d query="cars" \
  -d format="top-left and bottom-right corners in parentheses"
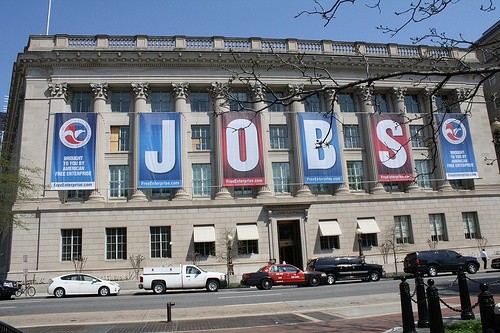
top-left (491, 258), bottom-right (500, 269)
top-left (240, 261), bottom-right (327, 290)
top-left (47, 273), bottom-right (121, 298)
top-left (0, 279), bottom-right (18, 300)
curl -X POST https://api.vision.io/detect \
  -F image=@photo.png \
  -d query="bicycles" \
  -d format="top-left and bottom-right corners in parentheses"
top-left (15, 280), bottom-right (36, 297)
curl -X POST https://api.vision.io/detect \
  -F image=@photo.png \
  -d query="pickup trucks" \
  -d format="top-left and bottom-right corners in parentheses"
top-left (138, 262), bottom-right (227, 294)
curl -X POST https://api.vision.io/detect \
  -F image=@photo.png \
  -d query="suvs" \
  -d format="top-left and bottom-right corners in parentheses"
top-left (306, 255), bottom-right (386, 285)
top-left (403, 249), bottom-right (480, 277)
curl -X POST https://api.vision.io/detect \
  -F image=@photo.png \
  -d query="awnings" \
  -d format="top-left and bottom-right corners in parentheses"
top-left (357, 219), bottom-right (380, 234)
top-left (236, 224), bottom-right (259, 240)
top-left (318, 221), bottom-right (342, 237)
top-left (194, 226), bottom-right (216, 242)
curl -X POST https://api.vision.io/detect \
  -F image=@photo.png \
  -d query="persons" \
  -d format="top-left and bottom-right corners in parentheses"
top-left (481, 249), bottom-right (490, 269)
